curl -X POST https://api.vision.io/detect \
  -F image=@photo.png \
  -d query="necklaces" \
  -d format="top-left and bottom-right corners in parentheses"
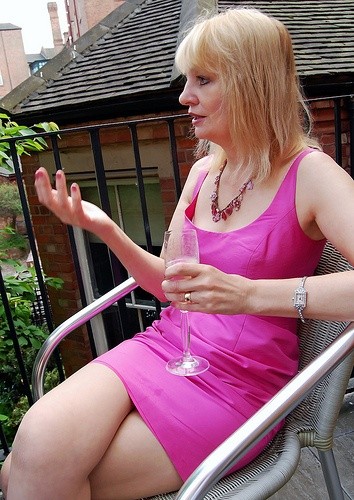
top-left (208, 158), bottom-right (261, 223)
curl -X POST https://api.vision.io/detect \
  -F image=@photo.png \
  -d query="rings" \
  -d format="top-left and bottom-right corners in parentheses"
top-left (182, 289), bottom-right (194, 306)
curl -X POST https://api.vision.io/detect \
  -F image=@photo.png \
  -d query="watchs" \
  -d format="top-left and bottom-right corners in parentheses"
top-left (292, 273), bottom-right (311, 328)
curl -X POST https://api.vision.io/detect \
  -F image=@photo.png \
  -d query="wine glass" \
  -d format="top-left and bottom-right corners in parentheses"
top-left (163, 228), bottom-right (210, 376)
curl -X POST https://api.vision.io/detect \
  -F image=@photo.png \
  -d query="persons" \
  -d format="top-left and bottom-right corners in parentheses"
top-left (0, 7), bottom-right (354, 500)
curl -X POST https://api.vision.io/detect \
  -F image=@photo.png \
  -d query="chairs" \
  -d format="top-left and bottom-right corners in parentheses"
top-left (31, 240), bottom-right (354, 500)
